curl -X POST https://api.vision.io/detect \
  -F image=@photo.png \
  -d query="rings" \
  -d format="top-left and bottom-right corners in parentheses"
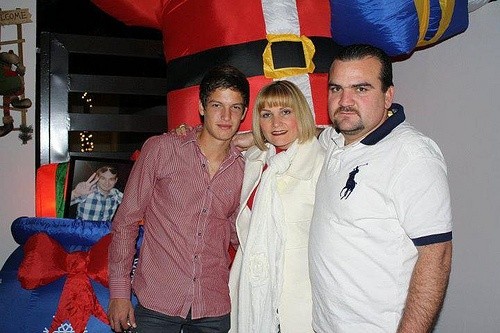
top-left (180, 125), bottom-right (185, 128)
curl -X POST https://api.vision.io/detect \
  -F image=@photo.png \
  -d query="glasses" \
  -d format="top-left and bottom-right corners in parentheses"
top-left (100, 167), bottom-right (117, 173)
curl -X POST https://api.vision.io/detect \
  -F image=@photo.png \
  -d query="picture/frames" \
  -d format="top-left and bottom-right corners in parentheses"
top-left (63, 155), bottom-right (135, 221)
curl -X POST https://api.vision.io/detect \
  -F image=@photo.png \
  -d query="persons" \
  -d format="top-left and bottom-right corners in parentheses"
top-left (232, 44), bottom-right (452, 333)
top-left (169, 81), bottom-right (324, 333)
top-left (71, 164), bottom-right (123, 221)
top-left (108, 66), bottom-right (245, 333)
